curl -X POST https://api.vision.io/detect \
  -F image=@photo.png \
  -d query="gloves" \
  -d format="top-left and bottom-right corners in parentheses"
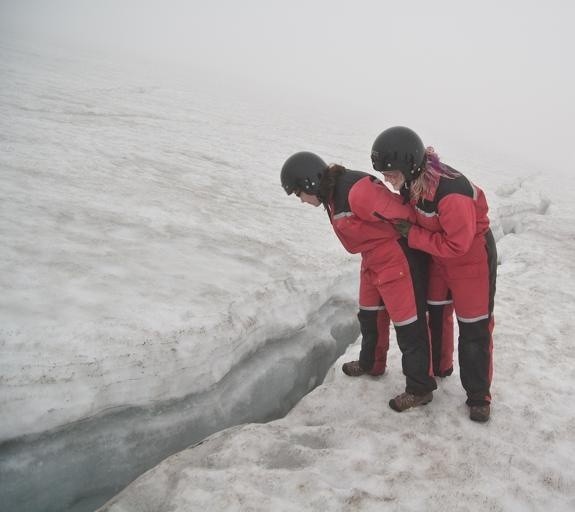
top-left (393, 221), bottom-right (412, 240)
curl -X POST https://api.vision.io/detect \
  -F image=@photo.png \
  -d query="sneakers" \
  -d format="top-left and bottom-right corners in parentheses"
top-left (389, 389), bottom-right (433, 412)
top-left (341, 360), bottom-right (386, 376)
top-left (470, 403), bottom-right (490, 421)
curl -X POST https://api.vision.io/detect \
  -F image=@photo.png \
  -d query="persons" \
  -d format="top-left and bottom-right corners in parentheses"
top-left (281, 151), bottom-right (437, 413)
top-left (370, 127), bottom-right (498, 420)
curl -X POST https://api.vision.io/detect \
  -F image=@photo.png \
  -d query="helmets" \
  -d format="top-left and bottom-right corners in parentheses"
top-left (370, 126), bottom-right (427, 185)
top-left (279, 151), bottom-right (329, 200)
